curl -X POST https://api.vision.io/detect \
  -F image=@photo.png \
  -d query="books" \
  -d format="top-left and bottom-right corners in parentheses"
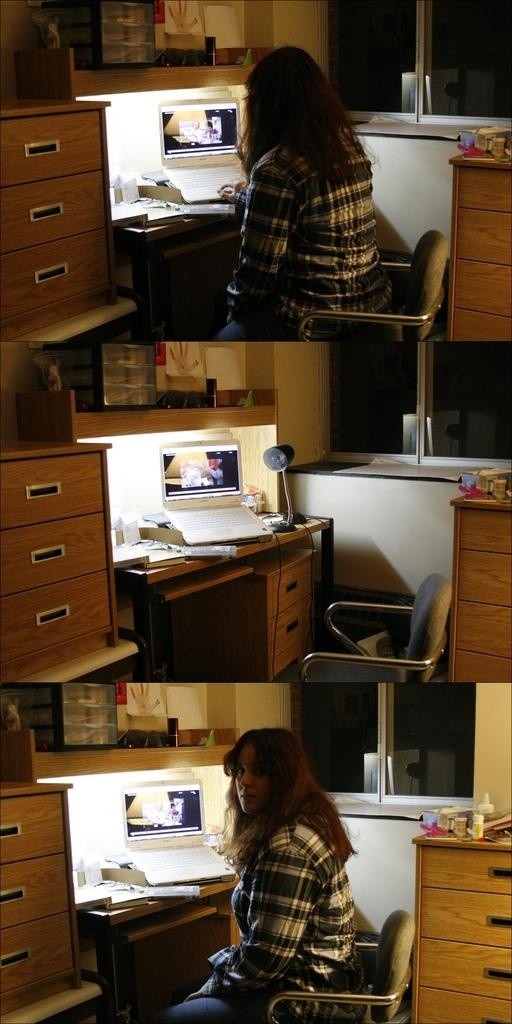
top-left (142, 549), bottom-right (187, 570)
top-left (112, 202), bottom-right (149, 227)
top-left (76, 887), bottom-right (113, 910)
top-left (183, 545), bottom-right (238, 561)
top-left (113, 544), bottom-right (149, 570)
top-left (180, 203), bottom-right (236, 218)
top-left (106, 893), bottom-right (150, 912)
top-left (144, 210), bottom-right (185, 228)
top-left (146, 885), bottom-right (200, 901)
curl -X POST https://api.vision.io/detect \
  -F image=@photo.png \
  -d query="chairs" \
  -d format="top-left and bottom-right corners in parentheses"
top-left (299, 229), bottom-right (450, 343)
top-left (265, 909), bottom-right (416, 1024)
top-left (305, 573), bottom-right (453, 682)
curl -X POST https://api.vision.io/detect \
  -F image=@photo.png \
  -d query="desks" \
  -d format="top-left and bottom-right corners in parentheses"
top-left (116, 511), bottom-right (335, 683)
top-left (21, 294), bottom-right (138, 343)
top-left (113, 200), bottom-right (241, 345)
top-left (1, 982), bottom-right (101, 1024)
top-left (78, 868), bottom-right (239, 1023)
top-left (23, 640), bottom-right (137, 682)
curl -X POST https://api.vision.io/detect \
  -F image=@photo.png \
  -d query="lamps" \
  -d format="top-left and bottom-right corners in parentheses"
top-left (263, 444), bottom-right (297, 533)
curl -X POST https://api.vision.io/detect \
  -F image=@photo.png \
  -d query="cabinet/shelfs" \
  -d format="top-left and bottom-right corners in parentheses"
top-left (1, 781), bottom-right (81, 1017)
top-left (446, 153), bottom-right (511, 341)
top-left (0, 443), bottom-right (120, 685)
top-left (6, 683), bottom-right (118, 752)
top-left (411, 831), bottom-right (512, 1023)
top-left (31, 342), bottom-right (157, 413)
top-left (448, 493), bottom-right (512, 682)
top-left (40, 0), bottom-right (157, 67)
top-left (13, 47), bottom-right (272, 200)
top-left (1, 728), bottom-right (240, 900)
top-left (0, 98), bottom-right (117, 342)
top-left (16, 390), bottom-right (280, 541)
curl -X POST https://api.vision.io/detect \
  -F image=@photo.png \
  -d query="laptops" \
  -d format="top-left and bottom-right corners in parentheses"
top-left (158, 97), bottom-right (249, 204)
top-left (120, 777), bottom-right (236, 884)
top-left (159, 439), bottom-right (274, 546)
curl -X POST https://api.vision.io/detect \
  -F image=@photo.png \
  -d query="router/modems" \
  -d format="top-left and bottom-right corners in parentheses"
top-left (142, 171), bottom-right (170, 186)
top-left (105, 853), bottom-right (134, 868)
top-left (143, 513), bottom-right (171, 527)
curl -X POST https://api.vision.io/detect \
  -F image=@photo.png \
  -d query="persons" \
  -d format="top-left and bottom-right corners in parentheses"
top-left (168, 0), bottom-right (198, 33)
top-left (129, 683), bottom-right (161, 714)
top-left (169, 342), bottom-right (200, 375)
top-left (204, 120), bottom-right (220, 139)
top-left (199, 459), bottom-right (224, 485)
top-left (211, 44), bottom-right (391, 342)
top-left (168, 803), bottom-right (179, 819)
top-left (160, 727), bottom-right (370, 1023)
top-left (184, 122), bottom-right (203, 138)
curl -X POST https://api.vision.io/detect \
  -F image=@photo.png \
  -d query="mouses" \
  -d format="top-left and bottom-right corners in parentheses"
top-left (283, 512), bottom-right (308, 524)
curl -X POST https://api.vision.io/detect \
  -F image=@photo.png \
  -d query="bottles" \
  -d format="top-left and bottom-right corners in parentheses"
top-left (168, 717), bottom-right (179, 747)
top-left (205, 36), bottom-right (216, 65)
top-left (473, 815), bottom-right (484, 840)
top-left (478, 792), bottom-right (494, 819)
top-left (495, 137), bottom-right (506, 161)
top-left (206, 379), bottom-right (217, 409)
top-left (506, 475), bottom-right (512, 492)
top-left (466, 813), bottom-right (473, 829)
top-left (505, 133), bottom-right (511, 150)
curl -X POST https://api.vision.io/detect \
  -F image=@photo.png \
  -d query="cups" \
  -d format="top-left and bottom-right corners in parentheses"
top-left (493, 479), bottom-right (506, 502)
top-left (455, 817), bottom-right (467, 839)
